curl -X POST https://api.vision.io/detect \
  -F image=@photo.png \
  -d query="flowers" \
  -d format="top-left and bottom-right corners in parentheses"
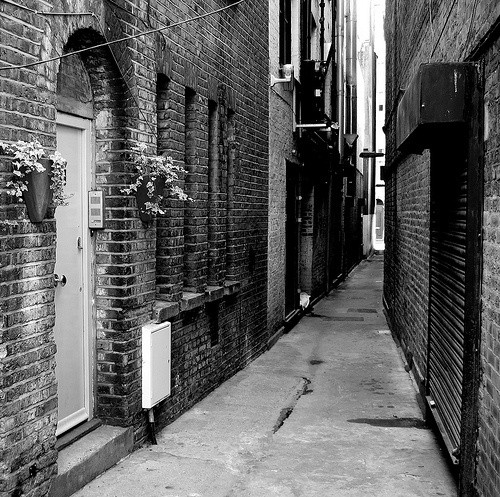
top-left (0, 139), bottom-right (75, 223)
top-left (118, 142), bottom-right (195, 219)
